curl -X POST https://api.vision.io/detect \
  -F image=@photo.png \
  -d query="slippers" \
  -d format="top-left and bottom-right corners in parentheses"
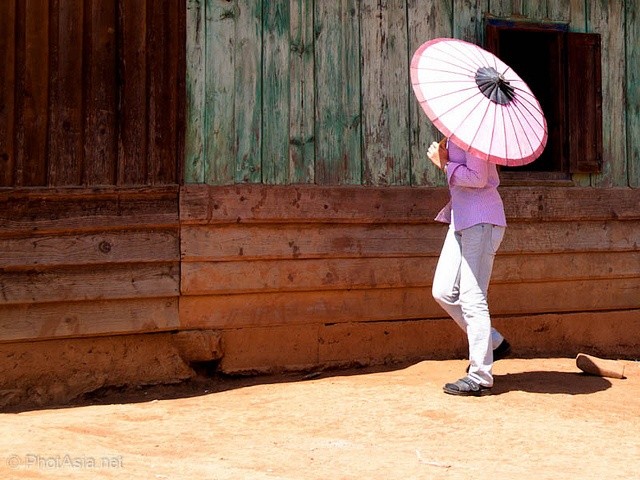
top-left (442, 377), bottom-right (497, 396)
top-left (466, 345), bottom-right (512, 373)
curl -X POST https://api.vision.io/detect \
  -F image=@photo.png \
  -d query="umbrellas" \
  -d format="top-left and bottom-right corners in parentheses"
top-left (410, 37), bottom-right (550, 167)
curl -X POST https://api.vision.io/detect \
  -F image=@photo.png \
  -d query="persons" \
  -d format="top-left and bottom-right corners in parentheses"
top-left (426, 139), bottom-right (509, 396)
